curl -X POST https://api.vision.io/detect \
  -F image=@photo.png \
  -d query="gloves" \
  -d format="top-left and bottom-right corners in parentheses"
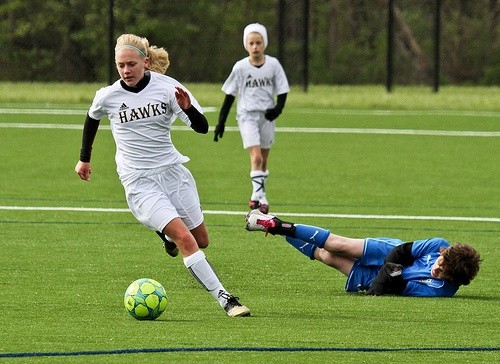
top-left (213, 124), bottom-right (225, 141)
top-left (265, 108), bottom-right (277, 122)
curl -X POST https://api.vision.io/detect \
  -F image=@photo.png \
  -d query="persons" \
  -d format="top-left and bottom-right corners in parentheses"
top-left (214, 23), bottom-right (290, 213)
top-left (246, 210), bottom-right (485, 296)
top-left (76, 33), bottom-right (250, 317)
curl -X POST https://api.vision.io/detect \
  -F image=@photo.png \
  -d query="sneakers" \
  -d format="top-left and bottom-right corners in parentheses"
top-left (156, 229), bottom-right (179, 257)
top-left (219, 292), bottom-right (250, 317)
top-left (249, 195), bottom-right (269, 214)
top-left (245, 210), bottom-right (278, 236)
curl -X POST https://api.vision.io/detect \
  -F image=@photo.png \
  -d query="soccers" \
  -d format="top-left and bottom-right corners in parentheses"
top-left (124, 278), bottom-right (168, 321)
